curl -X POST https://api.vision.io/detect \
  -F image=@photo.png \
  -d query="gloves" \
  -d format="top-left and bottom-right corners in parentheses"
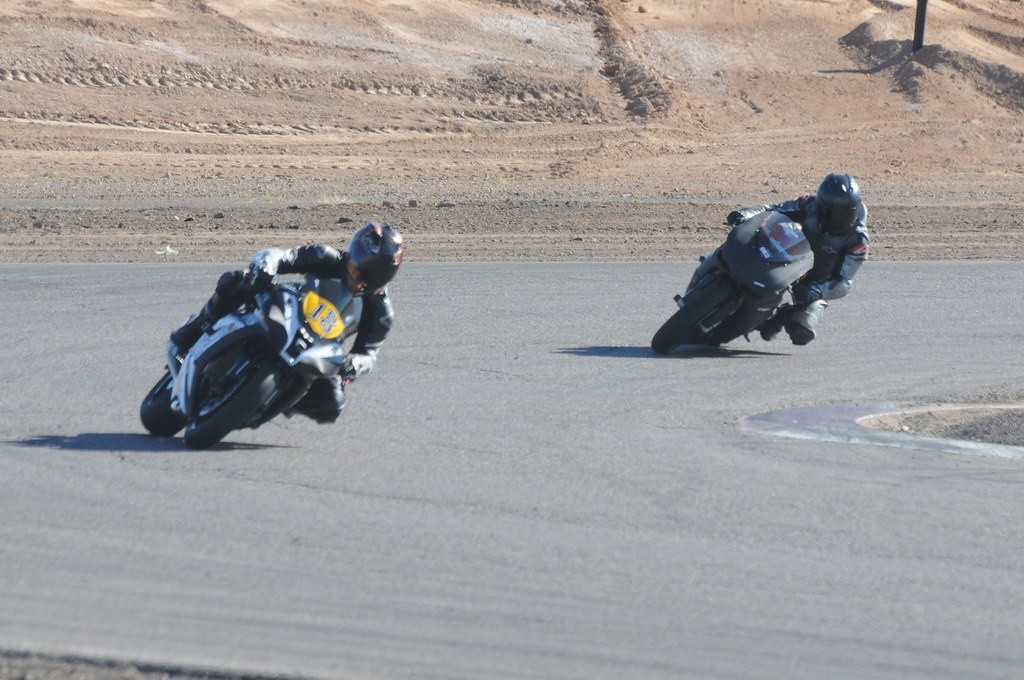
top-left (342, 357), bottom-right (355, 376)
top-left (245, 263), bottom-right (275, 291)
top-left (795, 283), bottom-right (824, 303)
top-left (726, 209), bottom-right (748, 225)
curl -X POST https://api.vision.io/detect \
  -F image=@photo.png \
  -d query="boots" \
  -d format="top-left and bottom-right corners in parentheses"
top-left (760, 301), bottom-right (796, 342)
top-left (171, 288), bottom-right (229, 353)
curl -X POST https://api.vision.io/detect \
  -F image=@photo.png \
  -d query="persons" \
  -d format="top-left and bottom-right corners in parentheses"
top-left (171, 221), bottom-right (402, 424)
top-left (727, 172), bottom-right (870, 346)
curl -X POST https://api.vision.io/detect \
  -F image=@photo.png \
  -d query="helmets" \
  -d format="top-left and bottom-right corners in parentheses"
top-left (814, 172), bottom-right (863, 235)
top-left (346, 221), bottom-right (403, 297)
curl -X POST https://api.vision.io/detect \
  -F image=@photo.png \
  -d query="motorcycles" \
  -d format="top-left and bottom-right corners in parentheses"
top-left (140, 261), bottom-right (363, 451)
top-left (652, 211), bottom-right (814, 354)
